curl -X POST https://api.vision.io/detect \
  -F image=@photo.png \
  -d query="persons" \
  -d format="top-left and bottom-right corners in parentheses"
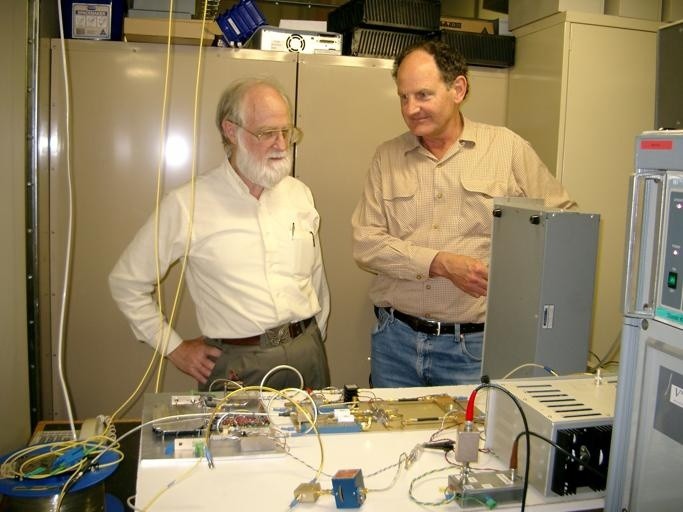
top-left (350, 40), bottom-right (579, 389)
top-left (108, 77), bottom-right (331, 391)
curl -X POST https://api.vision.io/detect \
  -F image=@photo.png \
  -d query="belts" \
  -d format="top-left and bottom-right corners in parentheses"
top-left (384, 306), bottom-right (484, 334)
top-left (215, 316), bottom-right (314, 347)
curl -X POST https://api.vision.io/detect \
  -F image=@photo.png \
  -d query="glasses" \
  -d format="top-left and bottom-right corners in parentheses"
top-left (227, 118), bottom-right (295, 143)
